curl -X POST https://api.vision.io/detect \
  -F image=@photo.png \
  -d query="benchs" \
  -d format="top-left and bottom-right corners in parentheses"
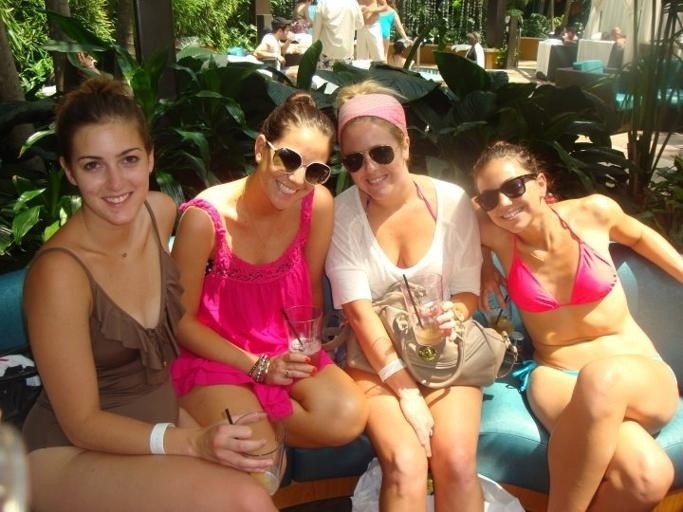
top-left (389, 41), bottom-right (504, 69)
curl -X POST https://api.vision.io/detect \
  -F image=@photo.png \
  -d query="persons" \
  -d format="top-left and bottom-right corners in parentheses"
top-left (169, 90), bottom-right (370, 496)
top-left (19, 72), bottom-right (276, 511)
top-left (251, 0), bottom-right (486, 98)
top-left (321, 79), bottom-right (487, 512)
top-left (464, 139), bottom-right (683, 512)
top-left (554, 22), bottom-right (626, 70)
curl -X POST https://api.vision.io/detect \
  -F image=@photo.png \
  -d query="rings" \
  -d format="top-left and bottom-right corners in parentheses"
top-left (285, 369), bottom-right (289, 377)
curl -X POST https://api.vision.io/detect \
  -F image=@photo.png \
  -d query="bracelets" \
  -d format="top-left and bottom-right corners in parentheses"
top-left (377, 358), bottom-right (406, 384)
top-left (149, 421), bottom-right (175, 456)
top-left (247, 353), bottom-right (272, 384)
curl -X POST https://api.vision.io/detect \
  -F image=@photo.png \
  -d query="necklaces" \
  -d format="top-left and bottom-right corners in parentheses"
top-left (114, 250), bottom-right (133, 259)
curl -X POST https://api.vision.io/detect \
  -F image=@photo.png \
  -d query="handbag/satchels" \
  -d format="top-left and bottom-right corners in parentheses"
top-left (345, 279), bottom-right (518, 390)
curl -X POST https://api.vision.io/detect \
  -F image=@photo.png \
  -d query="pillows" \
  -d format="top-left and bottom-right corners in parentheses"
top-left (572, 59), bottom-right (604, 73)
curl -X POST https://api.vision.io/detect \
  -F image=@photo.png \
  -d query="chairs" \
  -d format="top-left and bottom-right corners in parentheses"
top-left (556, 59), bottom-right (643, 131)
top-left (536, 38), bottom-right (624, 81)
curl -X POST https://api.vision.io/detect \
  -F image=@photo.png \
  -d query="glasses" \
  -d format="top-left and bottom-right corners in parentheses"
top-left (473, 173), bottom-right (538, 214)
top-left (339, 135), bottom-right (403, 173)
top-left (265, 139), bottom-right (332, 187)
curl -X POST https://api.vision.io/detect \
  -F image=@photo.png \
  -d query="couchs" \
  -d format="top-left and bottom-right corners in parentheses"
top-left (0, 237), bottom-right (683, 511)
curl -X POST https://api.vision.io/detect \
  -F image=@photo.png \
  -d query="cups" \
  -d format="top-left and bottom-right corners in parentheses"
top-left (480, 289), bottom-right (512, 337)
top-left (283, 305), bottom-right (322, 371)
top-left (400, 274), bottom-right (446, 346)
top-left (287, 32), bottom-right (296, 41)
top-left (232, 410), bottom-right (287, 495)
